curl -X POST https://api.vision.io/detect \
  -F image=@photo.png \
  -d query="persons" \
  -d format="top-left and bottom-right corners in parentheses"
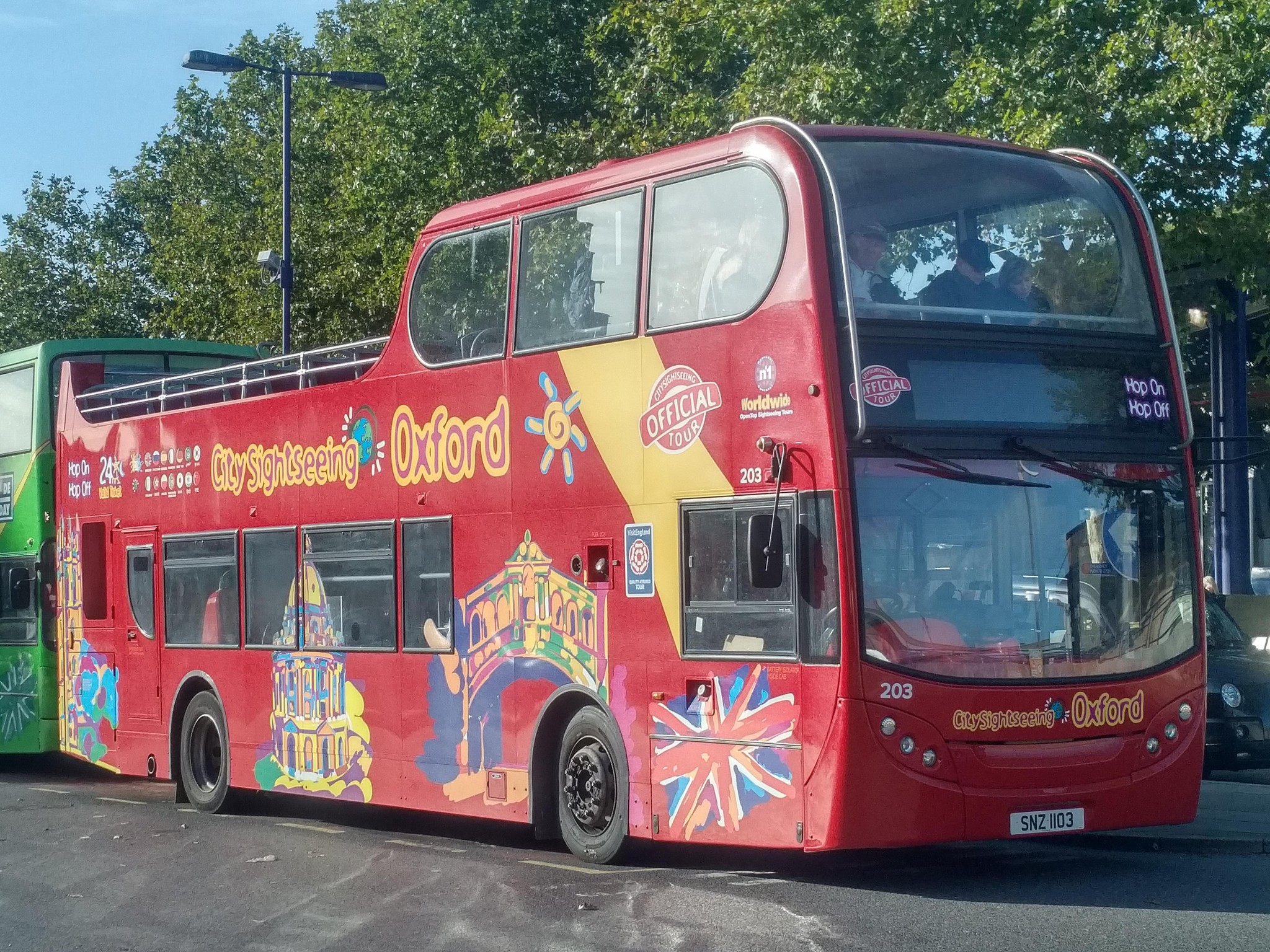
top-left (923, 239), bottom-right (1050, 313)
top-left (1203, 576), bottom-right (1219, 595)
top-left (844, 221), bottom-right (892, 302)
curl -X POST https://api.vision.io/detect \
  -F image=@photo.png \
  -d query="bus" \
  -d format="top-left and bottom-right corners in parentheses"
top-left (0, 341), bottom-right (278, 755)
top-left (53, 114), bottom-right (1213, 866)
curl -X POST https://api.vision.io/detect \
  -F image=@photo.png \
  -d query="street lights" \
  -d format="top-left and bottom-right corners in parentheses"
top-left (181, 50), bottom-right (389, 368)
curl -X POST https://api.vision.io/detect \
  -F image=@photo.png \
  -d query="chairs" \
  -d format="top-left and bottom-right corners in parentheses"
top-left (83, 326), bottom-right (504, 422)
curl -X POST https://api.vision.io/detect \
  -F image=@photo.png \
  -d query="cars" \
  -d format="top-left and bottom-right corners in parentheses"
top-left (1199, 582), bottom-right (1270, 760)
top-left (1009, 572), bottom-right (1120, 647)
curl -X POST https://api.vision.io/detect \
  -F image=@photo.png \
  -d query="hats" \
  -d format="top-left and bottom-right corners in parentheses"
top-left (958, 239), bottom-right (996, 272)
top-left (845, 220), bottom-right (889, 242)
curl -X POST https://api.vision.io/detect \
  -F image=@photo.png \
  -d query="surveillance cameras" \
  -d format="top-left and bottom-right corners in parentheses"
top-left (257, 251), bottom-right (296, 276)
